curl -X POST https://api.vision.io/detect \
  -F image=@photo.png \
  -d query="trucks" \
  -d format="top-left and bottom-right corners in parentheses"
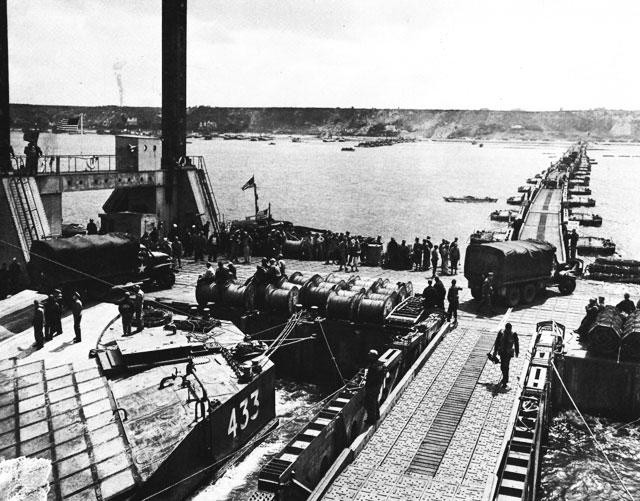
top-left (465, 238), bottom-right (575, 307)
top-left (28, 232), bottom-right (175, 305)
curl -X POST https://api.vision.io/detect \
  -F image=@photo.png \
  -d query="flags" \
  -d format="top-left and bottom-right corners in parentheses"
top-left (241, 177), bottom-right (256, 191)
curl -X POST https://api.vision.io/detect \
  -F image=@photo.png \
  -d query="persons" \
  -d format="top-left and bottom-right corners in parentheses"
top-left (422, 280), bottom-right (439, 315)
top-left (139, 215), bottom-right (461, 290)
top-left (363, 349), bottom-right (384, 425)
top-left (472, 272), bottom-right (500, 316)
top-left (573, 293), bottom-right (640, 345)
top-left (22, 142), bottom-right (45, 173)
top-left (7, 257), bottom-right (22, 293)
top-left (30, 300), bottom-right (44, 351)
top-left (492, 323), bottom-right (519, 388)
top-left (0, 263), bottom-right (8, 300)
top-left (42, 294), bottom-right (60, 341)
top-left (71, 292), bottom-right (83, 344)
top-left (568, 230), bottom-right (579, 259)
top-left (86, 219), bottom-right (97, 236)
top-left (446, 280), bottom-right (463, 324)
top-left (133, 284), bottom-right (144, 333)
top-left (432, 277), bottom-right (446, 315)
top-left (118, 292), bottom-right (135, 337)
top-left (51, 289), bottom-right (63, 336)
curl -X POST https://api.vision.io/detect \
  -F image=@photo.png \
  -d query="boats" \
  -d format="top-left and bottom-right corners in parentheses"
top-left (442, 173), bottom-right (542, 242)
top-left (568, 157), bottom-right (617, 254)
